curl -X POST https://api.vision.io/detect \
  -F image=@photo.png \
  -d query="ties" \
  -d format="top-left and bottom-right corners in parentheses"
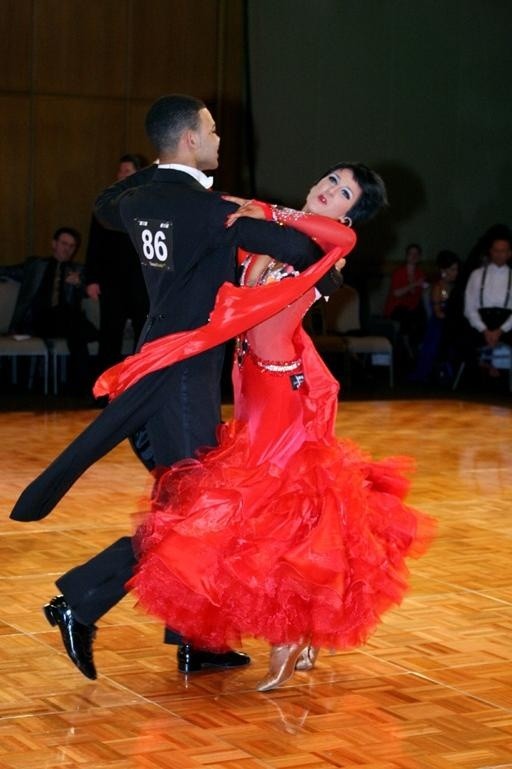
top-left (50, 261), bottom-right (63, 307)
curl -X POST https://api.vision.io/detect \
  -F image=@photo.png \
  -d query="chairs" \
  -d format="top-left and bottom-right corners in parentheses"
top-left (304, 285), bottom-right (395, 389)
top-left (0, 276), bottom-right (136, 398)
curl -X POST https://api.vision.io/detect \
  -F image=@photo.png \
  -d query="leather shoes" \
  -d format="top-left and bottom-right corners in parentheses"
top-left (42, 594), bottom-right (98, 681)
top-left (174, 642), bottom-right (251, 674)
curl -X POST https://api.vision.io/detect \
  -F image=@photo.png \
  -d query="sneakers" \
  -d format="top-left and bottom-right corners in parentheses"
top-left (295, 645), bottom-right (317, 673)
top-left (256, 632), bottom-right (307, 692)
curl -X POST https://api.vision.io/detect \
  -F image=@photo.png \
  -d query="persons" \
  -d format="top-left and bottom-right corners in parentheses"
top-left (74, 151), bottom-right (148, 411)
top-left (2, 226), bottom-right (96, 407)
top-left (43, 94), bottom-right (347, 681)
top-left (308, 233), bottom-right (511, 403)
top-left (220, 163), bottom-right (391, 690)
